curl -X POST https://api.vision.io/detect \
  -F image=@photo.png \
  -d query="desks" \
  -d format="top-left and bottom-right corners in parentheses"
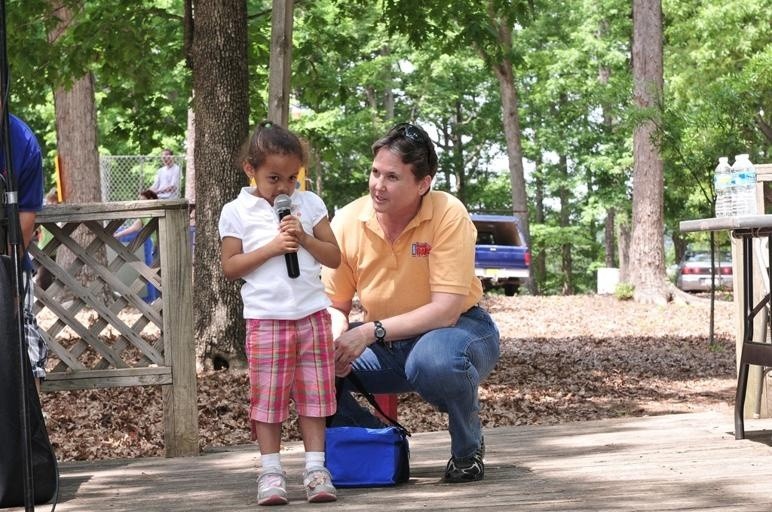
top-left (679, 214), bottom-right (772, 439)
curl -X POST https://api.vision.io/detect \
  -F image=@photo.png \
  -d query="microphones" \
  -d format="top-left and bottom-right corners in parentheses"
top-left (274, 193), bottom-right (300, 278)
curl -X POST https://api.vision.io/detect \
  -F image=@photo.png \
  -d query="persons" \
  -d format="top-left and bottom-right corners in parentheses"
top-left (319, 124), bottom-right (500, 483)
top-left (148, 149), bottom-right (183, 200)
top-left (219, 120), bottom-right (342, 505)
top-left (113, 190), bottom-right (160, 258)
top-left (3, 113), bottom-right (43, 316)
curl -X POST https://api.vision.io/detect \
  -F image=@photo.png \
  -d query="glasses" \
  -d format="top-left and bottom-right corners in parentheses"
top-left (389, 123), bottom-right (430, 164)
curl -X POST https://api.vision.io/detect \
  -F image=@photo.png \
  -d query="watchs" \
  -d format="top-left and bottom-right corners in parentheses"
top-left (373, 320), bottom-right (385, 344)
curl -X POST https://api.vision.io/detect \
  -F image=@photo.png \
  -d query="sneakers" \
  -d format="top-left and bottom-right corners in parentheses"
top-left (255, 468), bottom-right (289, 506)
top-left (302, 464), bottom-right (338, 503)
top-left (443, 434), bottom-right (486, 484)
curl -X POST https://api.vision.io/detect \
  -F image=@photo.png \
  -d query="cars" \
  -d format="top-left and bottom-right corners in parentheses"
top-left (674, 249), bottom-right (735, 294)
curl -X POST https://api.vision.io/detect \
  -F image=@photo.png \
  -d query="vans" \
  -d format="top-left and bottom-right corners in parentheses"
top-left (466, 208), bottom-right (533, 298)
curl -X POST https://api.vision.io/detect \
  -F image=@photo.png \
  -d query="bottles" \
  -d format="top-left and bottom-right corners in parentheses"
top-left (730, 154), bottom-right (758, 216)
top-left (713, 157), bottom-right (732, 218)
